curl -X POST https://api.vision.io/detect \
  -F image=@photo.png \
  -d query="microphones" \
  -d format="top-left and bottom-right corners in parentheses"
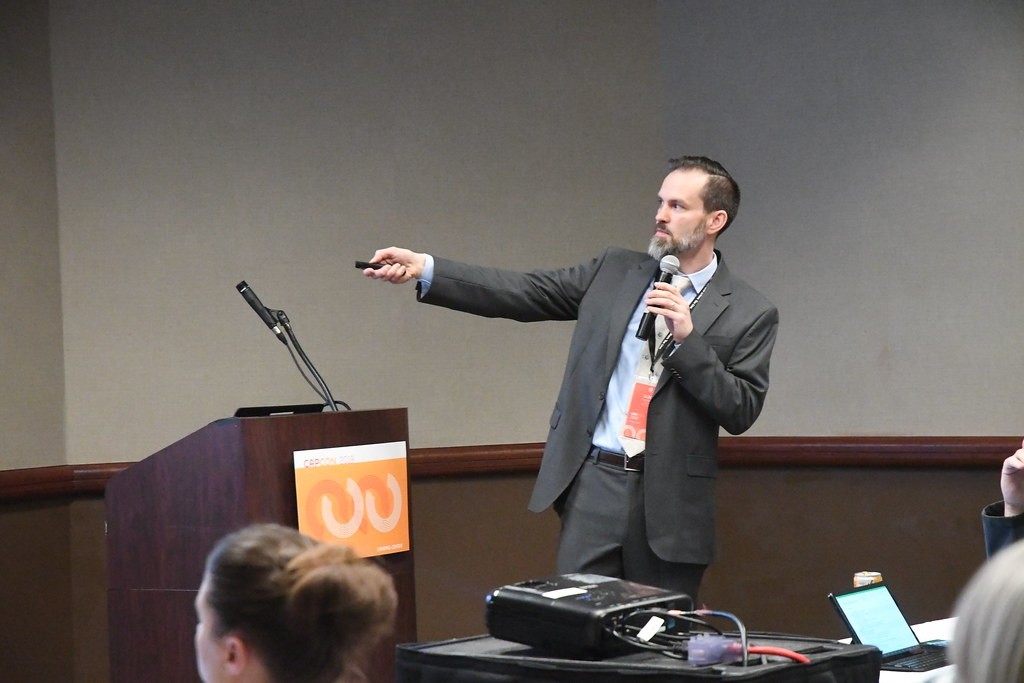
top-left (635, 255), bottom-right (680, 341)
top-left (235, 281), bottom-right (287, 345)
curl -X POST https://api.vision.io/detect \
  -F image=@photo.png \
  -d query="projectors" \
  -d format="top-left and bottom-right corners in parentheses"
top-left (485, 573), bottom-right (694, 661)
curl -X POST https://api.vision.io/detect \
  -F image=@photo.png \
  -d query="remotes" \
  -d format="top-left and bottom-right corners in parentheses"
top-left (353, 260), bottom-right (406, 276)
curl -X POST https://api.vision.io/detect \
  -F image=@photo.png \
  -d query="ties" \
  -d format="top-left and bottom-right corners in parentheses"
top-left (617, 273), bottom-right (692, 458)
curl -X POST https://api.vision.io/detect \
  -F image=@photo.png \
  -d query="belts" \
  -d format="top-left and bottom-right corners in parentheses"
top-left (587, 447), bottom-right (644, 471)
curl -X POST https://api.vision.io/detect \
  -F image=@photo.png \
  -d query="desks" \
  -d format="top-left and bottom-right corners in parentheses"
top-left (837, 616), bottom-right (957, 683)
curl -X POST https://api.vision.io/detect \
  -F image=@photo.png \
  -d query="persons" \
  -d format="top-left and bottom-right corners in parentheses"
top-left (980, 436), bottom-right (1024, 563)
top-left (950, 537), bottom-right (1024, 683)
top-left (355, 156), bottom-right (781, 624)
top-left (190, 520), bottom-right (401, 683)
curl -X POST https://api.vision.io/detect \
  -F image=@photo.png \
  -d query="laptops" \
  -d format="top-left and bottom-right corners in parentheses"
top-left (828, 581), bottom-right (952, 672)
